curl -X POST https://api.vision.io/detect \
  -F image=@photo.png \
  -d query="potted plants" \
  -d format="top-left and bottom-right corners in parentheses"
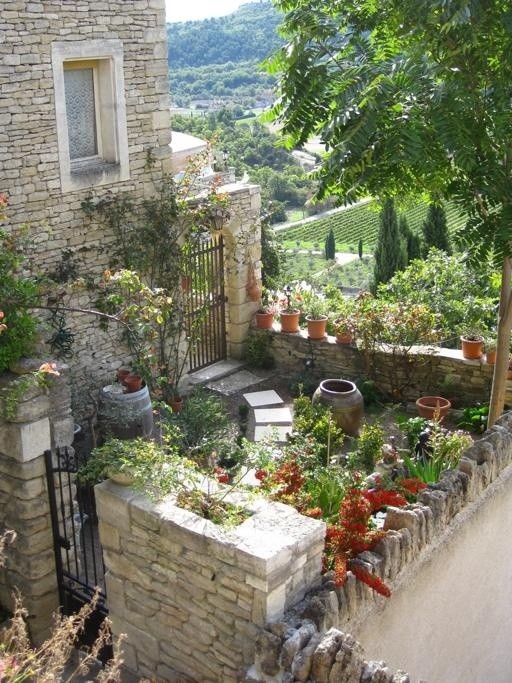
top-left (215, 441), bottom-right (240, 477)
top-left (167, 389), bottom-right (184, 415)
top-left (76, 434), bottom-right (190, 500)
top-left (279, 288), bottom-right (301, 333)
top-left (256, 291), bottom-right (274, 330)
top-left (482, 340), bottom-right (498, 364)
top-left (459, 315), bottom-right (485, 358)
top-left (332, 303), bottom-right (356, 346)
top-left (305, 298), bottom-right (329, 339)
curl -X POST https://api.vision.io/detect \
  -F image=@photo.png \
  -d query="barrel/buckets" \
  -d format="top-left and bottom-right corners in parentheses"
top-left (98, 383), bottom-right (153, 445)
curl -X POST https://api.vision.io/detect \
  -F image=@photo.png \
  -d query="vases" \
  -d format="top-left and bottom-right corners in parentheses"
top-left (416, 395), bottom-right (452, 419)
top-left (115, 368), bottom-right (142, 391)
